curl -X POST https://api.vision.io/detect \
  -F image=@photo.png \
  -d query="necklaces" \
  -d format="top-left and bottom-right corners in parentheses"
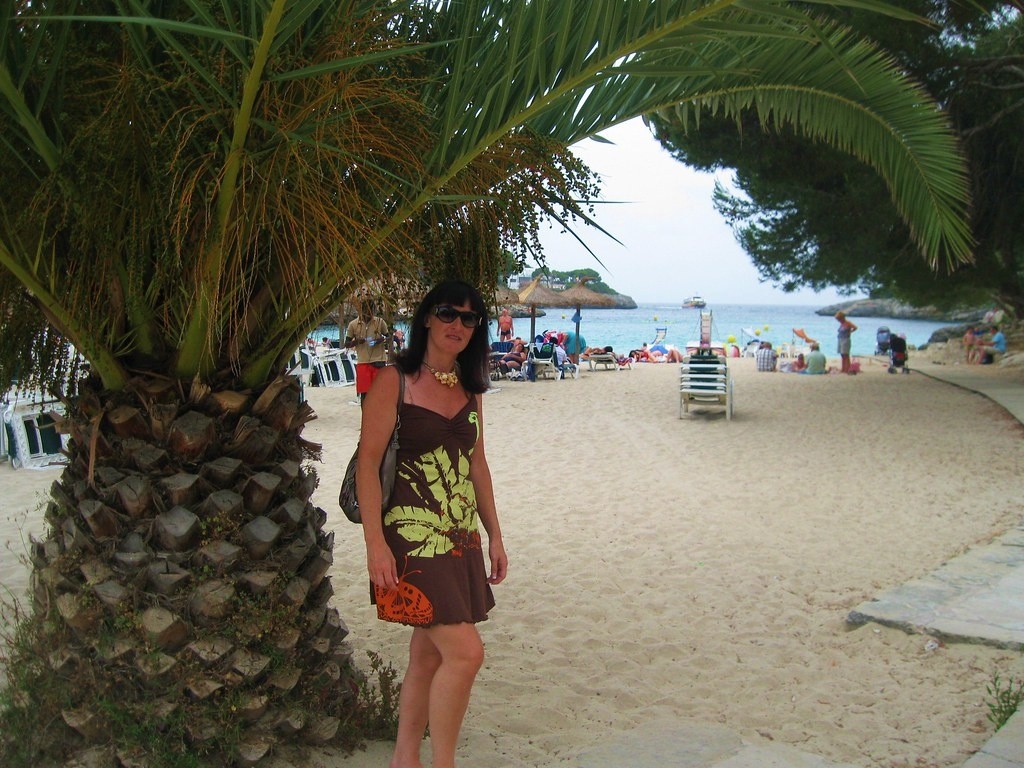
top-left (422, 360), bottom-right (459, 388)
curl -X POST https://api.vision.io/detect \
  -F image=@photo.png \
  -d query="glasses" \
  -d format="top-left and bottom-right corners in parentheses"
top-left (429, 304), bottom-right (482, 329)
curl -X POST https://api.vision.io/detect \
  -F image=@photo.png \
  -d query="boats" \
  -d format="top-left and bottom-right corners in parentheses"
top-left (681, 296), bottom-right (707, 309)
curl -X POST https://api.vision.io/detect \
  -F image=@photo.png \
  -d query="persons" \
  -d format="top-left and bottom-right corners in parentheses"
top-left (756, 342), bottom-right (779, 372)
top-left (306, 337), bottom-right (335, 349)
top-left (345, 299), bottom-right (391, 411)
top-left (383, 325), bottom-right (404, 361)
top-left (788, 345), bottom-right (832, 375)
top-left (495, 309), bottom-right (698, 378)
top-left (834, 311), bottom-right (857, 374)
top-left (354, 280), bottom-right (507, 768)
top-left (963, 304), bottom-right (1008, 365)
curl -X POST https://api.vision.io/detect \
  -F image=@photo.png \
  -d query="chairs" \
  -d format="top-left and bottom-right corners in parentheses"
top-left (0, 399), bottom-right (70, 470)
top-left (588, 355), bottom-right (632, 372)
top-left (488, 341), bottom-right (581, 383)
top-left (679, 348), bottom-right (735, 421)
top-left (284, 342), bottom-right (358, 388)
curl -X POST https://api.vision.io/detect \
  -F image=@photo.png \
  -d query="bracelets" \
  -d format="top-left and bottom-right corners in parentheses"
top-left (383, 335), bottom-right (387, 342)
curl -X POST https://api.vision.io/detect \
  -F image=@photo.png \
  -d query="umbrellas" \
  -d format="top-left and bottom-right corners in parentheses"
top-left (346, 273), bottom-right (427, 327)
top-left (516, 274), bottom-right (567, 345)
top-left (561, 275), bottom-right (617, 365)
top-left (477, 283), bottom-right (518, 307)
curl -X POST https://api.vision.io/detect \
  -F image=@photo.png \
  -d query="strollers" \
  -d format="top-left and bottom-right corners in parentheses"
top-left (887, 332), bottom-right (911, 374)
top-left (875, 326), bottom-right (892, 355)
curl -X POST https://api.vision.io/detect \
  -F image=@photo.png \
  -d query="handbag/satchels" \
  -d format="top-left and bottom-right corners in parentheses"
top-left (339, 365), bottom-right (405, 524)
top-left (561, 358), bottom-right (575, 370)
top-left (848, 358), bottom-right (860, 373)
